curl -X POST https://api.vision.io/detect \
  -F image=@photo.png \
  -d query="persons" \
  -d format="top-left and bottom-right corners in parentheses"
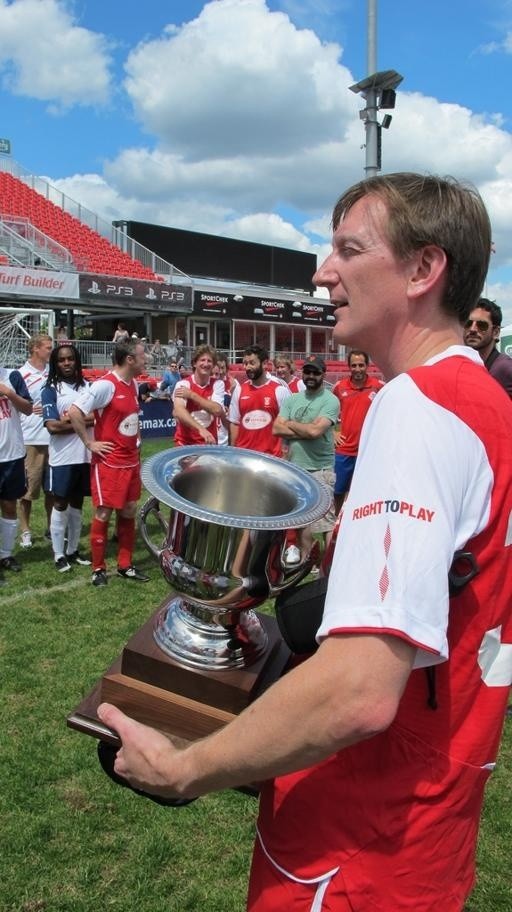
top-left (463, 298), bottom-right (512, 398)
top-left (1, 325), bottom-right (386, 586)
top-left (95, 170), bottom-right (512, 912)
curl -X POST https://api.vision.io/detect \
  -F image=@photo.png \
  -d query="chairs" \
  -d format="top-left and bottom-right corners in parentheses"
top-left (0, 170), bottom-right (165, 282)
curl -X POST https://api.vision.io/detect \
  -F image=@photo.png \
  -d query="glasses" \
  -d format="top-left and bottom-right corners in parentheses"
top-left (303, 369), bottom-right (323, 376)
top-left (465, 319), bottom-right (496, 331)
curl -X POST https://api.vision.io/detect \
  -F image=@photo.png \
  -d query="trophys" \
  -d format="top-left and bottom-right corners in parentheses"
top-left (67, 443), bottom-right (335, 798)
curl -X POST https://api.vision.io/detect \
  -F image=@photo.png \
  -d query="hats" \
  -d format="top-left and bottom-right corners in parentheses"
top-left (302, 354), bottom-right (326, 372)
top-left (148, 378), bottom-right (157, 391)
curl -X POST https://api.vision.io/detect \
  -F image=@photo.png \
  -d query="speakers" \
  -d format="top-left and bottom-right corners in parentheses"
top-left (381, 114), bottom-right (392, 128)
top-left (379, 89), bottom-right (397, 109)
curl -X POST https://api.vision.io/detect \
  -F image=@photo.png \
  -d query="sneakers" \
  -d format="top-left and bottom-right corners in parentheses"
top-left (285, 546), bottom-right (301, 565)
top-left (19, 530), bottom-right (33, 548)
top-left (55, 555), bottom-right (72, 574)
top-left (92, 568), bottom-right (108, 588)
top-left (311, 561), bottom-right (322, 574)
top-left (115, 563), bottom-right (150, 582)
top-left (44, 529), bottom-right (68, 542)
top-left (66, 552), bottom-right (93, 566)
top-left (2, 557), bottom-right (23, 572)
